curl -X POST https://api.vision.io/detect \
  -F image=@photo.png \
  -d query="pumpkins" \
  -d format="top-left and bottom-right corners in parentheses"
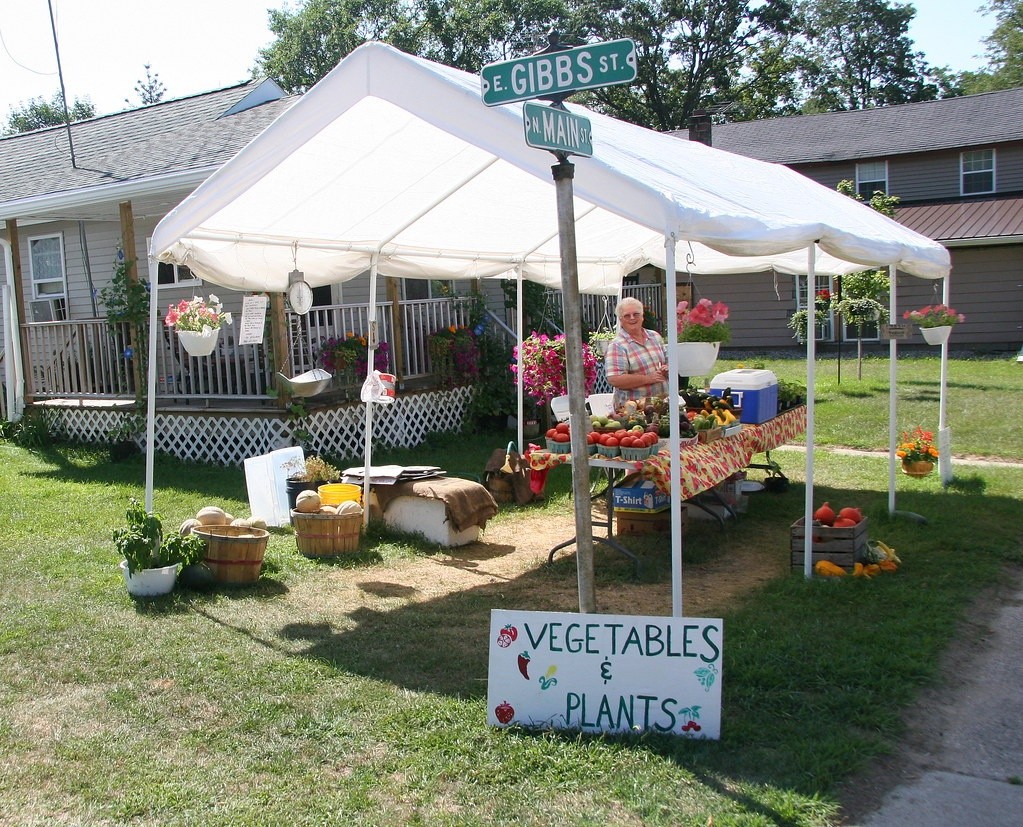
top-left (296, 490), bottom-right (363, 514)
top-left (179, 507), bottom-right (266, 537)
top-left (816, 540), bottom-right (901, 578)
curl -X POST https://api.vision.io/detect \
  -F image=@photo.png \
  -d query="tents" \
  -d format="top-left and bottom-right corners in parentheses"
top-left (145, 41), bottom-right (952, 616)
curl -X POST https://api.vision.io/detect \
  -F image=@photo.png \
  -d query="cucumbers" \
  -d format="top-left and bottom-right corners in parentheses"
top-left (692, 414), bottom-right (719, 430)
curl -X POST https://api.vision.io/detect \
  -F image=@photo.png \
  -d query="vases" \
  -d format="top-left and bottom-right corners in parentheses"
top-left (523, 423), bottom-right (540, 440)
top-left (176, 327), bottom-right (220, 357)
top-left (815, 302), bottom-right (830, 311)
top-left (765, 477), bottom-right (789, 494)
top-left (663, 341), bottom-right (721, 378)
top-left (901, 459), bottom-right (934, 475)
top-left (918, 325), bottom-right (952, 346)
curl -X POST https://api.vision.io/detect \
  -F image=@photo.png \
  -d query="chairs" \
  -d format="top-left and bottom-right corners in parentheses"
top-left (161, 318), bottom-right (228, 405)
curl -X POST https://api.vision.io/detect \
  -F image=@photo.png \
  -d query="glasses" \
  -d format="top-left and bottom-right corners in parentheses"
top-left (618, 313), bottom-right (642, 319)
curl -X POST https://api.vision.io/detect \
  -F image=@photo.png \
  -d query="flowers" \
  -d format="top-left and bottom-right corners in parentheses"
top-left (164, 293), bottom-right (234, 338)
top-left (676, 297), bottom-right (737, 348)
top-left (896, 424), bottom-right (938, 468)
top-left (316, 331), bottom-right (392, 404)
top-left (510, 331), bottom-right (597, 406)
top-left (425, 325), bottom-right (485, 391)
top-left (815, 289), bottom-right (831, 308)
top-left (903, 304), bottom-right (966, 328)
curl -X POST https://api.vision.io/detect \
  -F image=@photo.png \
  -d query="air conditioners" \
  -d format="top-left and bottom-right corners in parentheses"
top-left (28, 299), bottom-right (59, 323)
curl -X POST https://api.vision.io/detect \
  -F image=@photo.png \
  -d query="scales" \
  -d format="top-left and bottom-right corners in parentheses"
top-left (275, 269), bottom-right (332, 396)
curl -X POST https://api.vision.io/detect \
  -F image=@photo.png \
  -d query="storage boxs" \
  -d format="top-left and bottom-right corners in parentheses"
top-left (596, 443), bottom-right (621, 459)
top-left (657, 436), bottom-right (670, 447)
top-left (651, 443), bottom-right (658, 455)
top-left (709, 368), bottom-right (777, 424)
top-left (681, 502), bottom-right (732, 522)
top-left (545, 436), bottom-right (571, 454)
top-left (382, 495), bottom-right (480, 548)
top-left (587, 444), bottom-right (598, 456)
top-left (698, 427), bottom-right (722, 444)
top-left (619, 446), bottom-right (651, 461)
top-left (721, 424), bottom-right (741, 438)
top-left (789, 515), bottom-right (868, 576)
top-left (719, 495), bottom-right (750, 514)
top-left (680, 434), bottom-right (698, 446)
top-left (719, 478), bottom-right (743, 498)
top-left (594, 427), bottom-right (617, 434)
top-left (612, 472), bottom-right (671, 513)
top-left (684, 407), bottom-right (742, 418)
top-left (617, 507), bottom-right (688, 539)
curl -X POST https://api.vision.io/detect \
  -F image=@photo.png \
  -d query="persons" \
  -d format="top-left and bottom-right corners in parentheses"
top-left (606, 297), bottom-right (669, 482)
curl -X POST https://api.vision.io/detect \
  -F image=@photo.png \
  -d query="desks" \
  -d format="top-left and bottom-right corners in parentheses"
top-left (529, 404), bottom-right (808, 575)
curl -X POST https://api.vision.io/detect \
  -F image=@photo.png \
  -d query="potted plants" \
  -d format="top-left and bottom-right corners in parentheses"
top-left (587, 327), bottom-right (617, 360)
top-left (280, 454), bottom-right (344, 527)
top-left (787, 309), bottom-right (828, 344)
top-left (113, 497), bottom-right (215, 596)
top-left (104, 417), bottom-right (136, 464)
top-left (777, 379), bottom-right (808, 413)
top-left (220, 336), bottom-right (235, 356)
top-left (830, 269), bottom-right (891, 336)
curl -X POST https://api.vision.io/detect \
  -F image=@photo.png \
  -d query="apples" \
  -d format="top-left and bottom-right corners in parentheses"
top-left (585, 414), bottom-right (658, 448)
top-left (546, 423), bottom-right (570, 442)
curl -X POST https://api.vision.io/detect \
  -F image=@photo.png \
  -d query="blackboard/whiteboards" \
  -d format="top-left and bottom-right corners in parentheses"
top-left (486, 608), bottom-right (722, 738)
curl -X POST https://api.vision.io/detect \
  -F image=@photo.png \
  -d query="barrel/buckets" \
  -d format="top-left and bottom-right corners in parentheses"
top-left (190, 524), bottom-right (270, 585)
top-left (291, 507), bottom-right (362, 555)
top-left (317, 481), bottom-right (362, 508)
